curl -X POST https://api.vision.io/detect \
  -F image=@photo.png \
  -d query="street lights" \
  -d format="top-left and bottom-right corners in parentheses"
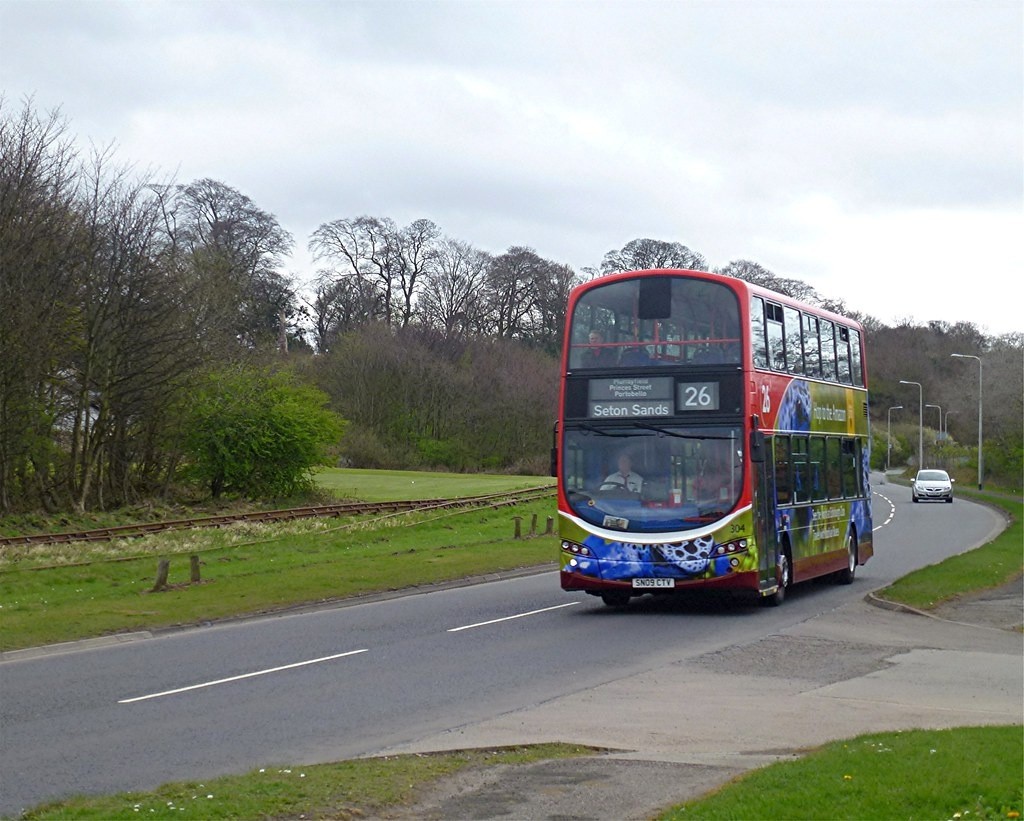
top-left (886, 406), bottom-right (903, 467)
top-left (925, 405), bottom-right (942, 441)
top-left (899, 381), bottom-right (924, 472)
top-left (950, 354), bottom-right (983, 490)
top-left (945, 410), bottom-right (959, 440)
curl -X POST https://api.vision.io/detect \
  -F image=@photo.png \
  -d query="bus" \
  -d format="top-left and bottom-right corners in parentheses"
top-left (546, 269), bottom-right (874, 608)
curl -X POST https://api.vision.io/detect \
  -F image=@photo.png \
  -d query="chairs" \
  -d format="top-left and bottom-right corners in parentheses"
top-left (776, 476), bottom-right (860, 504)
top-left (607, 457), bottom-right (648, 478)
top-left (621, 345), bottom-right (742, 364)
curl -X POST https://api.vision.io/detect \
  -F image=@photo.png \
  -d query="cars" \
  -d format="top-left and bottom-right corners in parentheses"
top-left (910, 469), bottom-right (955, 503)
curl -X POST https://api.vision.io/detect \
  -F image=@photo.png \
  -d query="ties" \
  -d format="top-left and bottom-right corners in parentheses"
top-left (594, 349), bottom-right (600, 357)
top-left (619, 474), bottom-right (630, 491)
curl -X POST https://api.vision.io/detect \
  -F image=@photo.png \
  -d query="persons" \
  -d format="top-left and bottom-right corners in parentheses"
top-left (600, 454), bottom-right (642, 494)
top-left (580, 330), bottom-right (616, 367)
